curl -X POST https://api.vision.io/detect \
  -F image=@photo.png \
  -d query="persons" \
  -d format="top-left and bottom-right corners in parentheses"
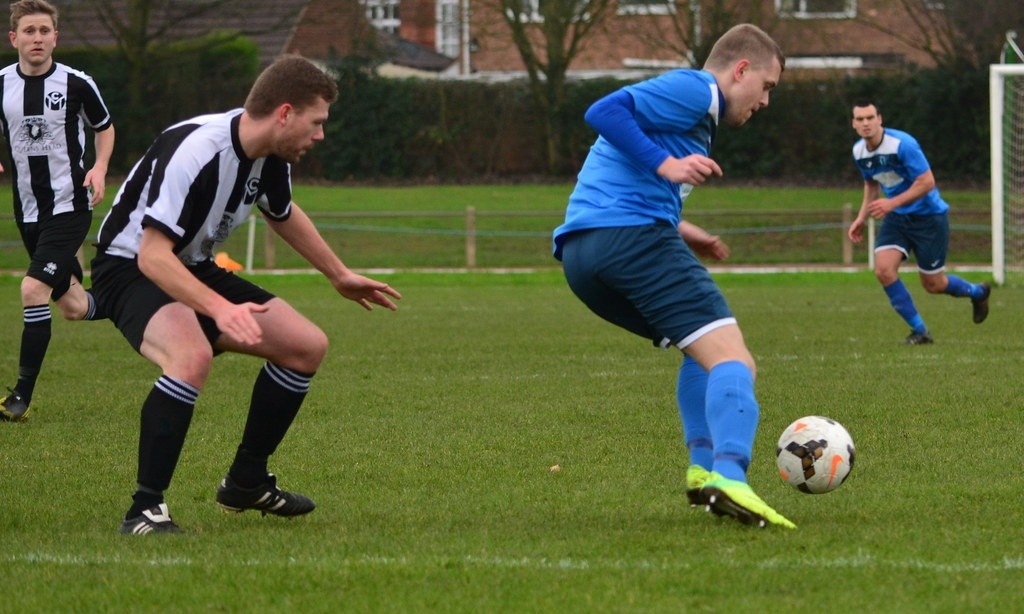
top-left (90, 57), bottom-right (402, 536)
top-left (848, 96), bottom-right (991, 345)
top-left (552, 21), bottom-right (800, 532)
top-left (0, 0), bottom-right (111, 423)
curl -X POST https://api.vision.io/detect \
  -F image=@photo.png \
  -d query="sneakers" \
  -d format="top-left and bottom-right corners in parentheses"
top-left (699, 471), bottom-right (797, 531)
top-left (903, 331), bottom-right (933, 345)
top-left (0, 386), bottom-right (31, 422)
top-left (216, 473), bottom-right (316, 517)
top-left (120, 503), bottom-right (184, 536)
top-left (971, 282), bottom-right (991, 324)
top-left (686, 465), bottom-right (711, 507)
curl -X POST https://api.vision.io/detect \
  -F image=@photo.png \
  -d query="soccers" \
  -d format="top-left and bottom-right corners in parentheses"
top-left (774, 414), bottom-right (858, 496)
